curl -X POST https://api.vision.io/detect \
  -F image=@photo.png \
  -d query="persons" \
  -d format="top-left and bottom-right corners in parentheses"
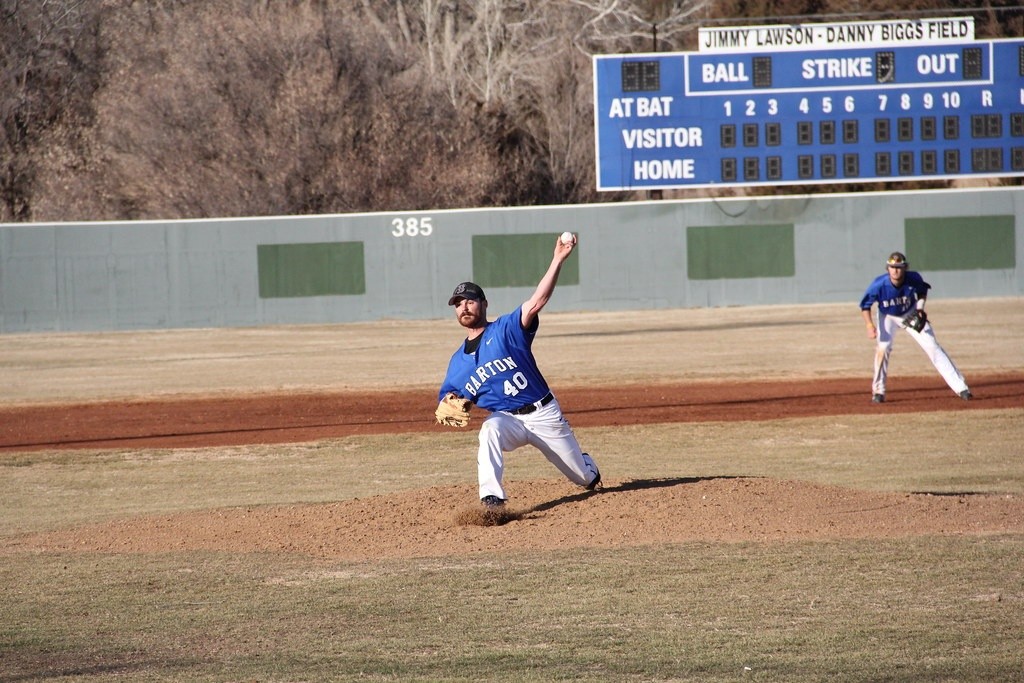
top-left (431, 230), bottom-right (604, 509)
top-left (859, 251), bottom-right (974, 403)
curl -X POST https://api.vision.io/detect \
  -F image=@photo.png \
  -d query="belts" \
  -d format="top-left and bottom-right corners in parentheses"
top-left (510, 392), bottom-right (554, 415)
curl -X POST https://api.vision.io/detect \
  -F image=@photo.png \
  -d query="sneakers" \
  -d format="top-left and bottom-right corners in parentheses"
top-left (480, 495), bottom-right (504, 510)
top-left (960, 390), bottom-right (972, 401)
top-left (581, 452), bottom-right (604, 491)
top-left (871, 394), bottom-right (885, 403)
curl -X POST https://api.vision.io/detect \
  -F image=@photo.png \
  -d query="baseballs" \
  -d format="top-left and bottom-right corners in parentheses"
top-left (560, 231), bottom-right (574, 244)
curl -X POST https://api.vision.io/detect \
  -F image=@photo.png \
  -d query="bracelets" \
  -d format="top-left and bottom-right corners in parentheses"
top-left (866, 322), bottom-right (875, 328)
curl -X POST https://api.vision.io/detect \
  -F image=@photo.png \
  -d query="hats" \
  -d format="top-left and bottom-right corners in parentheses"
top-left (448, 282), bottom-right (486, 305)
top-left (886, 252), bottom-right (908, 268)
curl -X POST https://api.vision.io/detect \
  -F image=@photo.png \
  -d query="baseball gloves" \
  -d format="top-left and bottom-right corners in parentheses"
top-left (435, 391), bottom-right (473, 428)
top-left (900, 308), bottom-right (927, 334)
